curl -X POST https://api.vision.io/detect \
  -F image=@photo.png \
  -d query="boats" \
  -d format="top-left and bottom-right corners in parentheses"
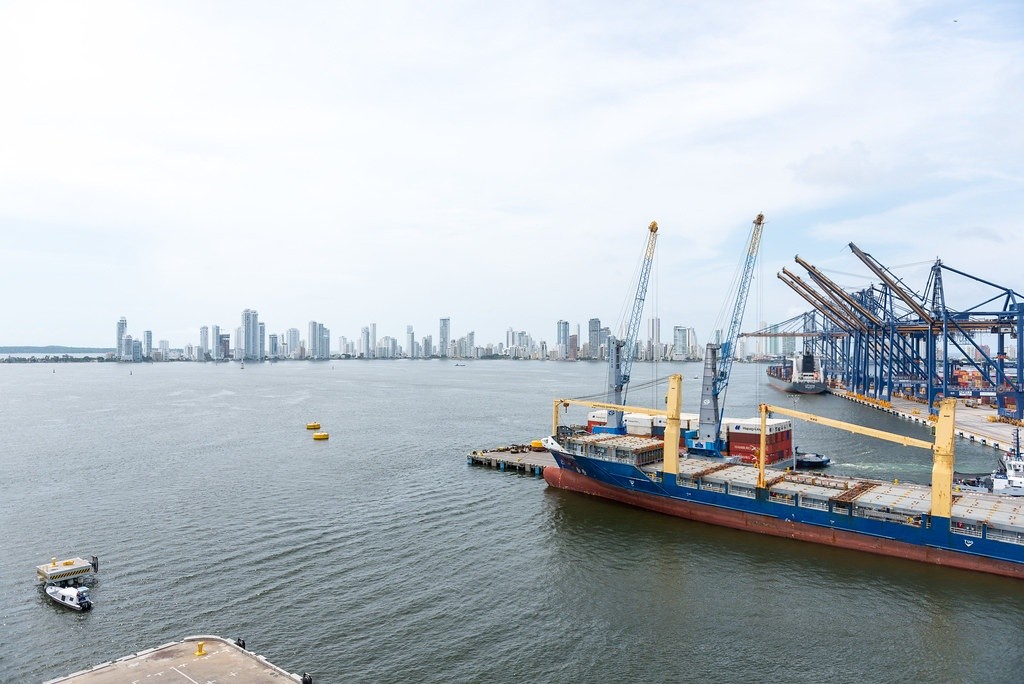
top-left (793, 447), bottom-right (829, 468)
top-left (953, 428), bottom-right (1024, 496)
top-left (767, 349), bottom-right (826, 395)
top-left (569, 409), bottom-right (796, 471)
top-left (46, 584), bottom-right (94, 612)
top-left (542, 374), bottom-right (1024, 578)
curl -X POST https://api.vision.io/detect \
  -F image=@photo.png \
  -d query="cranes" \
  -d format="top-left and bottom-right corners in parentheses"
top-left (586, 219), bottom-right (658, 434)
top-left (683, 210), bottom-right (766, 457)
